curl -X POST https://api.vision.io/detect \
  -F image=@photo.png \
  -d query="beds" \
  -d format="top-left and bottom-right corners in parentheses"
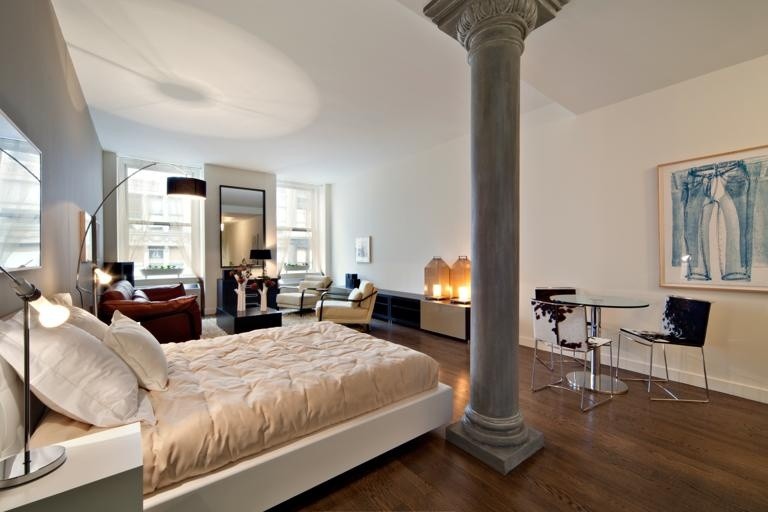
top-left (0, 291), bottom-right (454, 512)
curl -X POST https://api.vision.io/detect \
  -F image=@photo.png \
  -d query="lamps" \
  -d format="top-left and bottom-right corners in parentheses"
top-left (75, 160), bottom-right (208, 283)
top-left (0, 265), bottom-right (70, 488)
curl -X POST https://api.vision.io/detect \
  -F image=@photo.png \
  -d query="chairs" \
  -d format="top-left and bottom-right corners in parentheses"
top-left (89, 279), bottom-right (201, 344)
top-left (275, 274), bottom-right (378, 333)
top-left (616, 295), bottom-right (713, 403)
top-left (529, 285), bottom-right (613, 411)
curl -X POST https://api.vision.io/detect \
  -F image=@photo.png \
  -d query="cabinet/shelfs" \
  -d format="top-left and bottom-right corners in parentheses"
top-left (332, 285), bottom-right (470, 344)
top-left (217, 278), bottom-right (277, 307)
top-left (103, 262), bottom-right (134, 289)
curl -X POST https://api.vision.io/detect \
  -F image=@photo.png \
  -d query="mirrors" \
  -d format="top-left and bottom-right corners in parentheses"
top-left (218, 184), bottom-right (266, 269)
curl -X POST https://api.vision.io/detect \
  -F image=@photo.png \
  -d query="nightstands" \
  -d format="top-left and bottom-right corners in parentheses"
top-left (0, 422), bottom-right (145, 512)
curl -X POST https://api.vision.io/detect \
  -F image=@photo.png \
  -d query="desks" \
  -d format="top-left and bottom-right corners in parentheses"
top-left (549, 293), bottom-right (649, 395)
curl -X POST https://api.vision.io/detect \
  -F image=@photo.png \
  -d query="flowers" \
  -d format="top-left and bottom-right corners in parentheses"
top-left (227, 257), bottom-right (275, 289)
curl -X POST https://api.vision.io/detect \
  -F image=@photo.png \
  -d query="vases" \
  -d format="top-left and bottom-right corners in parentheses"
top-left (256, 282), bottom-right (269, 311)
top-left (232, 274), bottom-right (249, 312)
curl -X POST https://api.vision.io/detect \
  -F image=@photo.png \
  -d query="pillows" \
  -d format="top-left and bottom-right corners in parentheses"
top-left (0, 295), bottom-right (171, 428)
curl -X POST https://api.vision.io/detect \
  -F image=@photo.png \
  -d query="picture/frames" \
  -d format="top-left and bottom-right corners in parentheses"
top-left (655, 142), bottom-right (766, 293)
top-left (354, 235), bottom-right (371, 263)
